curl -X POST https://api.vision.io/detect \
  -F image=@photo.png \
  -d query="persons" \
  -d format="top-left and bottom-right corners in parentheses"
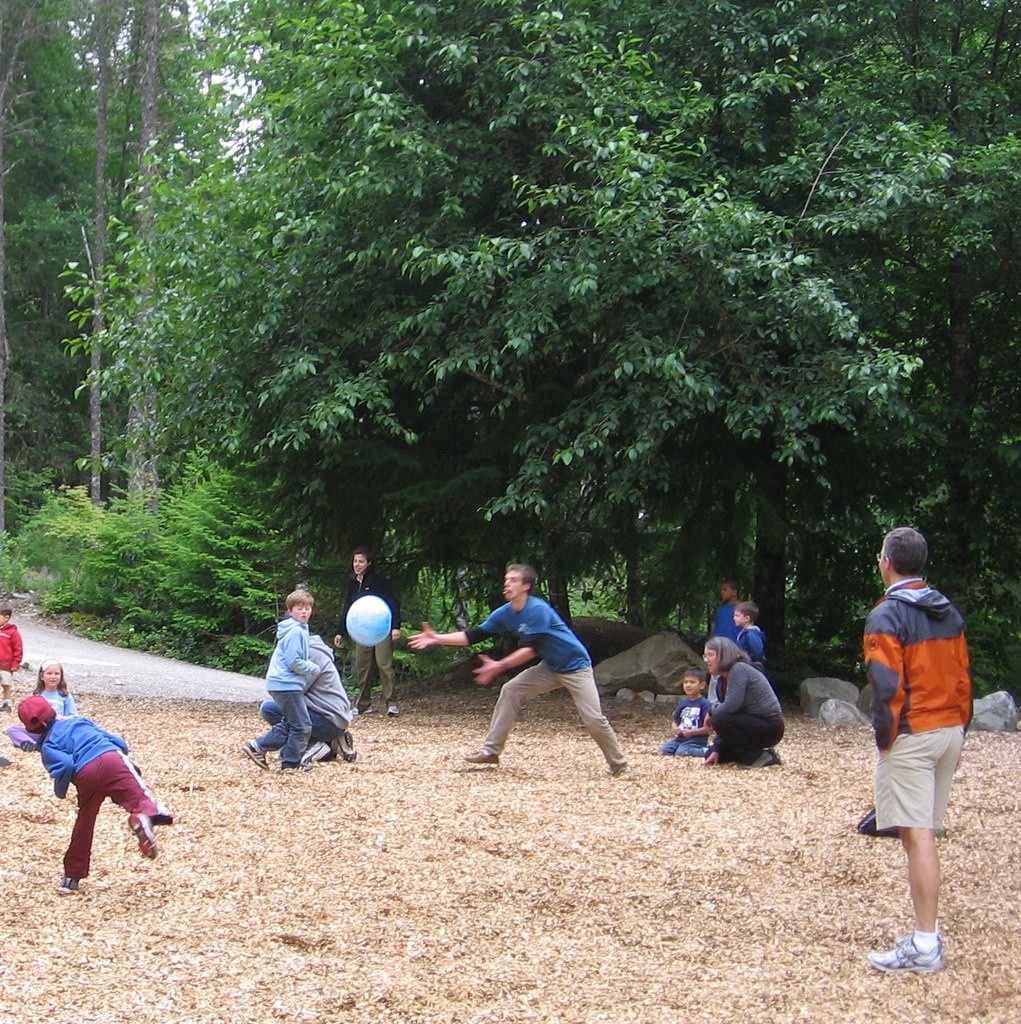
top-left (261, 635), bottom-right (356, 765)
top-left (0, 606), bottom-right (23, 713)
top-left (7, 659), bottom-right (78, 751)
top-left (733, 601), bottom-right (766, 662)
top-left (713, 578), bottom-right (743, 645)
top-left (407, 565), bottom-right (629, 775)
top-left (242, 591), bottom-right (320, 772)
top-left (334, 546), bottom-right (402, 715)
top-left (703, 636), bottom-right (785, 768)
top-left (660, 666), bottom-right (712, 756)
top-left (863, 527), bottom-right (974, 970)
top-left (17, 696), bottom-right (173, 895)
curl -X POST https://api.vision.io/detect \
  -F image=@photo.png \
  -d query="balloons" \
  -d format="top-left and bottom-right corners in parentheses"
top-left (345, 596), bottom-right (392, 646)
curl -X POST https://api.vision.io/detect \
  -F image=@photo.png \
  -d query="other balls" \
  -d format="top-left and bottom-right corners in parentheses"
top-left (346, 595), bottom-right (392, 647)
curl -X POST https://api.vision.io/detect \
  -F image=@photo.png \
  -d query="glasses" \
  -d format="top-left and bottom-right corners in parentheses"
top-left (702, 651), bottom-right (718, 661)
top-left (876, 552), bottom-right (886, 562)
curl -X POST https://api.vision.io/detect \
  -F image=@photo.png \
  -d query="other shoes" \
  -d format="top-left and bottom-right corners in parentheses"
top-left (0, 699), bottom-right (15, 713)
top-left (740, 748), bottom-right (782, 769)
top-left (20, 740), bottom-right (38, 751)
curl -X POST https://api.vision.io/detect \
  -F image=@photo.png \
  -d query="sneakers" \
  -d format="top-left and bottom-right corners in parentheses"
top-left (895, 918), bottom-right (944, 953)
top-left (351, 705), bottom-right (374, 714)
top-left (242, 744), bottom-right (268, 771)
top-left (866, 935), bottom-right (944, 975)
top-left (128, 814), bottom-right (156, 859)
top-left (301, 740), bottom-right (330, 765)
top-left (282, 763), bottom-right (312, 773)
top-left (465, 753), bottom-right (499, 764)
top-left (58, 876), bottom-right (79, 894)
top-left (386, 706), bottom-right (400, 715)
top-left (331, 731), bottom-right (357, 763)
top-left (610, 761), bottom-right (627, 778)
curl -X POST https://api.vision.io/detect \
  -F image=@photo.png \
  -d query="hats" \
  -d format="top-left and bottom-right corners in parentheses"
top-left (18, 696), bottom-right (52, 729)
